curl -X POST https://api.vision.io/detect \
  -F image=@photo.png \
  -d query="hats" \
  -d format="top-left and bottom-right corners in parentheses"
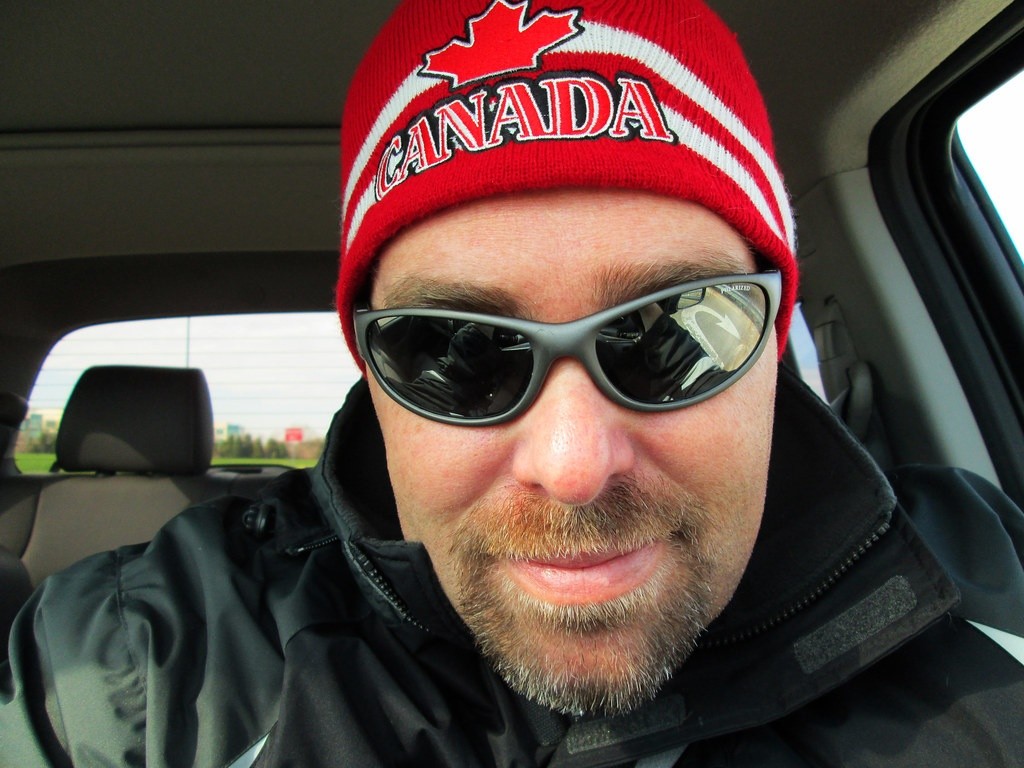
top-left (337, 0), bottom-right (798, 382)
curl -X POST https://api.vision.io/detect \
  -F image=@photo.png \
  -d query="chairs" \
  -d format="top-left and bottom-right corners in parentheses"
top-left (0, 364), bottom-right (281, 591)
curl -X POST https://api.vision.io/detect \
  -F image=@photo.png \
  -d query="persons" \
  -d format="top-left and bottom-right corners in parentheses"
top-left (0, 0), bottom-right (1024, 768)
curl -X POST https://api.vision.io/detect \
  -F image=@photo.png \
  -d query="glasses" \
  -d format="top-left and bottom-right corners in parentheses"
top-left (352, 268), bottom-right (780, 427)
